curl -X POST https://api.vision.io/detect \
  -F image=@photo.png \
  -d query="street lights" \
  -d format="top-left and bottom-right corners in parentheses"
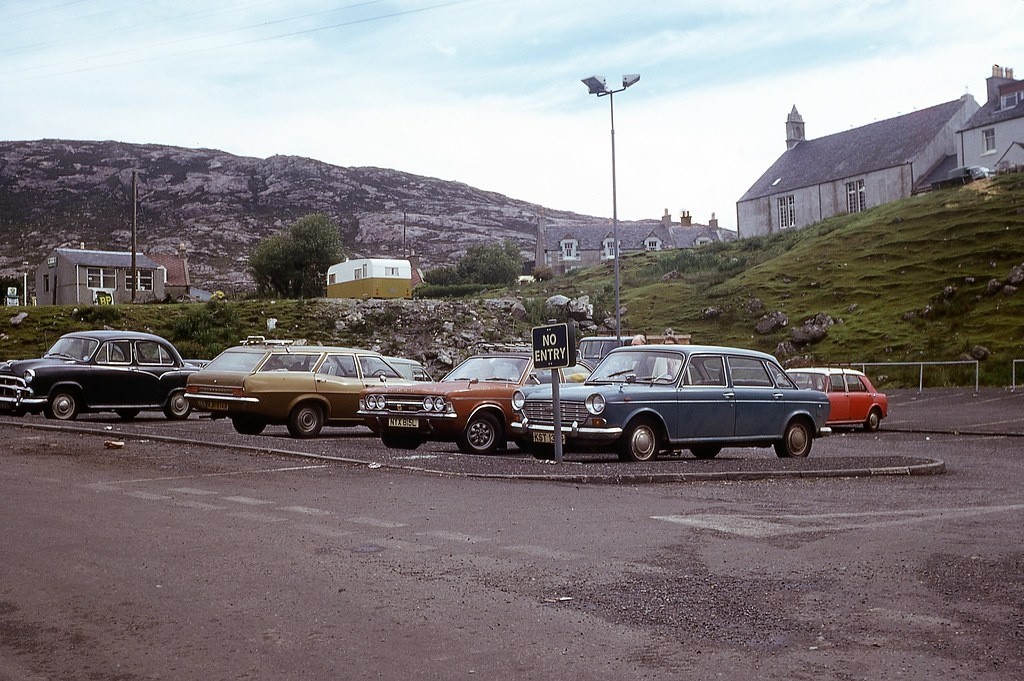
top-left (578, 72), bottom-right (642, 348)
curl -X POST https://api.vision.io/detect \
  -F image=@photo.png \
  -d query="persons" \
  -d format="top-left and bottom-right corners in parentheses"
top-left (631, 334), bottom-right (656, 377)
top-left (652, 336), bottom-right (682, 457)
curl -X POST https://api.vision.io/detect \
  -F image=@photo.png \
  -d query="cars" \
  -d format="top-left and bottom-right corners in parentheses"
top-left (359, 349), bottom-right (601, 454)
top-left (182, 359), bottom-right (213, 369)
top-left (0, 331), bottom-right (201, 423)
top-left (508, 344), bottom-right (831, 459)
top-left (182, 338), bottom-right (407, 440)
top-left (575, 335), bottom-right (693, 376)
top-left (374, 356), bottom-right (433, 384)
top-left (774, 368), bottom-right (888, 430)
